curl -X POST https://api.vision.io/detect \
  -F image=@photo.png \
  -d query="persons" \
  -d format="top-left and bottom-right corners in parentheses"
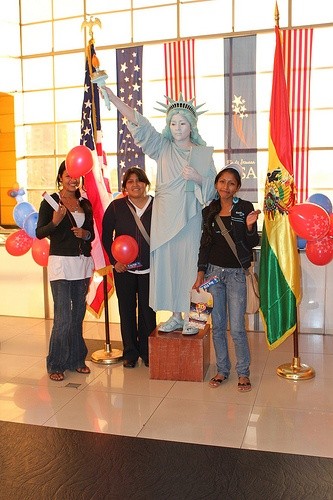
top-left (94, 86), bottom-right (219, 334)
top-left (35, 160), bottom-right (95, 381)
top-left (192, 168), bottom-right (262, 392)
top-left (101, 167), bottom-right (153, 368)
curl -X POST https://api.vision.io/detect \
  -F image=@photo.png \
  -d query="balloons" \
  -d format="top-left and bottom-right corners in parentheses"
top-left (32, 236), bottom-right (50, 266)
top-left (7, 183), bottom-right (25, 197)
top-left (113, 192), bottom-right (128, 200)
top-left (111, 235), bottom-right (139, 265)
top-left (13, 202), bottom-right (35, 229)
top-left (297, 193), bottom-right (333, 266)
top-left (288, 203), bottom-right (331, 241)
top-left (66, 146), bottom-right (94, 179)
top-left (23, 212), bottom-right (39, 239)
top-left (6, 230), bottom-right (33, 256)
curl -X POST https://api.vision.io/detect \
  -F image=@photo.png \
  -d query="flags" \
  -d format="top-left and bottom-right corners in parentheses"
top-left (257, 25), bottom-right (305, 351)
top-left (115, 34), bottom-right (258, 205)
top-left (78, 37), bottom-right (116, 321)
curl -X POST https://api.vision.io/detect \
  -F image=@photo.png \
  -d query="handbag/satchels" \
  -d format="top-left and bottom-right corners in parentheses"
top-left (245, 273), bottom-right (259, 314)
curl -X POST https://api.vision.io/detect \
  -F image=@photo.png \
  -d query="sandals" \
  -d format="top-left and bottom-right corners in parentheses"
top-left (208, 376), bottom-right (228, 388)
top-left (48, 372), bottom-right (64, 381)
top-left (238, 376), bottom-right (251, 392)
top-left (76, 367), bottom-right (90, 374)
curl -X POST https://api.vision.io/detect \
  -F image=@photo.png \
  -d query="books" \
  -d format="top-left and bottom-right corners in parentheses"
top-left (185, 146), bottom-right (214, 191)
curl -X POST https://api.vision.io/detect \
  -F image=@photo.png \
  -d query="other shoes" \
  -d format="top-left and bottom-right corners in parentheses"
top-left (145, 360), bottom-right (149, 367)
top-left (123, 360), bottom-right (137, 368)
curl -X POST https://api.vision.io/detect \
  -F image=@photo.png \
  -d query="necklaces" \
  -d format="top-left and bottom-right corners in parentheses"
top-left (60, 190), bottom-right (79, 215)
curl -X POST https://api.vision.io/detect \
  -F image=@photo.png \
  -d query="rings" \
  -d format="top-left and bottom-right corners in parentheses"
top-left (125, 266), bottom-right (128, 269)
